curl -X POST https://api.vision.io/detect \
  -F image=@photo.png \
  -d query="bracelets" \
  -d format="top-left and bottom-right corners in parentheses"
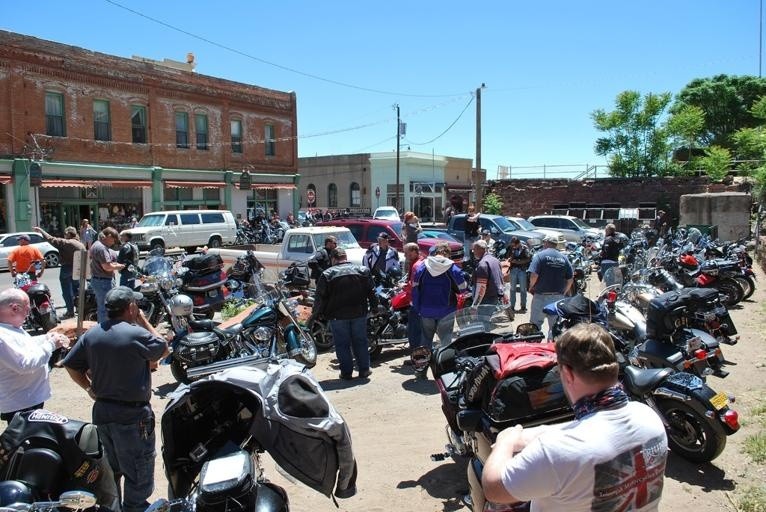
top-left (85, 384), bottom-right (94, 391)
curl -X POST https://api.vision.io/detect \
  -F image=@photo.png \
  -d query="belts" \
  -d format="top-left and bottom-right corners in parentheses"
top-left (96, 397), bottom-right (149, 408)
top-left (93, 276), bottom-right (111, 280)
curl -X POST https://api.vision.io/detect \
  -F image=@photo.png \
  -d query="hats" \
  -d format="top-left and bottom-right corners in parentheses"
top-left (543, 234), bottom-right (559, 243)
top-left (16, 235), bottom-right (29, 241)
top-left (104, 286), bottom-right (145, 312)
top-left (377, 232), bottom-right (389, 239)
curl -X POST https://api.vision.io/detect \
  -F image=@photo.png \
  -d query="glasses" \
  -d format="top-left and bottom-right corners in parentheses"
top-left (9, 303), bottom-right (31, 316)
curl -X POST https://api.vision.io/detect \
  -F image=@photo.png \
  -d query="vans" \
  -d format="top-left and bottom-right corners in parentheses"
top-left (117, 208), bottom-right (244, 251)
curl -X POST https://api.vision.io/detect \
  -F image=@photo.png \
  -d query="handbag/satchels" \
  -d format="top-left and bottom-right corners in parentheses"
top-left (180, 253), bottom-right (224, 276)
top-left (284, 265), bottom-right (311, 289)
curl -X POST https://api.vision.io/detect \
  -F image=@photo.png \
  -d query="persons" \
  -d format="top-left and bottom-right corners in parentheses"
top-left (62, 284), bottom-right (170, 512)
top-left (479, 319), bottom-right (671, 512)
top-left (31, 225), bottom-right (89, 321)
top-left (73, 207), bottom-right (665, 378)
top-left (0, 287), bottom-right (72, 425)
top-left (7, 234), bottom-right (48, 282)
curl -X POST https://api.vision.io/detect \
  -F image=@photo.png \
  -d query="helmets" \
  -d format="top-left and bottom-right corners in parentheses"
top-left (170, 294), bottom-right (193, 316)
top-left (388, 266), bottom-right (402, 281)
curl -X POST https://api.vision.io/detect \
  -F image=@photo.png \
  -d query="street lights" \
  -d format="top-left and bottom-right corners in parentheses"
top-left (389, 102), bottom-right (401, 209)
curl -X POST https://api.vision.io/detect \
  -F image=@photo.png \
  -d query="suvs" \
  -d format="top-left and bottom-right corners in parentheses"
top-left (287, 203), bottom-right (599, 256)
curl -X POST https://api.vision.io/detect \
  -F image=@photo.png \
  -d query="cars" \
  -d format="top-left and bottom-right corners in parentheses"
top-left (1, 231), bottom-right (63, 271)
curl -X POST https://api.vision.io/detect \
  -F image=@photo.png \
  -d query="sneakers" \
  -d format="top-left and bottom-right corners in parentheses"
top-left (123, 501), bottom-right (152, 512)
top-left (518, 307), bottom-right (527, 314)
top-left (63, 312), bottom-right (74, 319)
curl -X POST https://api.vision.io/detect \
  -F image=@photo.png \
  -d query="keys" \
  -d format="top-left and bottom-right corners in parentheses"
top-left (138, 420), bottom-right (150, 441)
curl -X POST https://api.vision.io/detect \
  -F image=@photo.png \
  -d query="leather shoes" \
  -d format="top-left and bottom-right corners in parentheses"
top-left (339, 374), bottom-right (353, 381)
top-left (359, 370), bottom-right (373, 379)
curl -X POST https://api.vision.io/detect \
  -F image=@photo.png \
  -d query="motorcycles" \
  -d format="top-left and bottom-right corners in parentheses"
top-left (247, 217), bottom-right (294, 241)
top-left (413, 292), bottom-right (740, 512)
top-left (0, 411), bottom-right (117, 512)
top-left (146, 369), bottom-right (345, 512)
top-left (101, 251), bottom-right (412, 374)
top-left (21, 283), bottom-right (58, 333)
top-left (500, 245), bottom-right (604, 289)
top-left (628, 214), bottom-right (756, 327)
